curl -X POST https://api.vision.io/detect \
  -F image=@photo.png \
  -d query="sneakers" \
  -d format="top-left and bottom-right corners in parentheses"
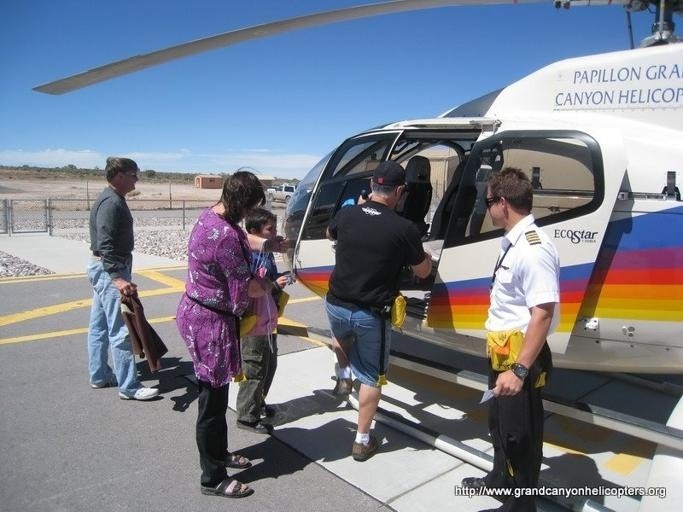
top-left (90, 374), bottom-right (118, 388)
top-left (261, 403), bottom-right (276, 416)
top-left (236, 419), bottom-right (273, 434)
top-left (333, 378), bottom-right (352, 394)
top-left (352, 431), bottom-right (383, 461)
top-left (118, 385), bottom-right (158, 399)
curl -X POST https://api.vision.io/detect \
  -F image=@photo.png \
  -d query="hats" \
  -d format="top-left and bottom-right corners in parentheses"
top-left (372, 160), bottom-right (405, 186)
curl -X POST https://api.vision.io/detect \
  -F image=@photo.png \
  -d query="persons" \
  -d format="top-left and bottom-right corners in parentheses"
top-left (462, 167), bottom-right (559, 510)
top-left (176, 172), bottom-right (266, 499)
top-left (88, 158), bottom-right (159, 400)
top-left (235, 208), bottom-right (295, 432)
top-left (325, 161), bottom-right (433, 462)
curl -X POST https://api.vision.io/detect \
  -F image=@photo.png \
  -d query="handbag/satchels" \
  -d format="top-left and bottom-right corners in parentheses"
top-left (487, 328), bottom-right (523, 372)
top-left (389, 296), bottom-right (408, 329)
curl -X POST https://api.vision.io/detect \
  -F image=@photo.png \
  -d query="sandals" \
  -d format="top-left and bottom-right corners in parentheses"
top-left (201, 476), bottom-right (252, 497)
top-left (227, 452), bottom-right (250, 468)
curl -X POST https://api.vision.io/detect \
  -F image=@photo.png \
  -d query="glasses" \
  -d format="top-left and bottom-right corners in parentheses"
top-left (483, 196), bottom-right (499, 208)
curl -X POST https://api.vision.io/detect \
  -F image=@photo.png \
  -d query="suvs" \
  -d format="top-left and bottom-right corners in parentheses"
top-left (267, 186), bottom-right (308, 203)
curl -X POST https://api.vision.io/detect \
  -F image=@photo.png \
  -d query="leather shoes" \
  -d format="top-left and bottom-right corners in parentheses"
top-left (462, 477), bottom-right (488, 494)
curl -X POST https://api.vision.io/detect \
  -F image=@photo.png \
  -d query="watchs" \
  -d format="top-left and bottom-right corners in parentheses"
top-left (509, 363), bottom-right (529, 379)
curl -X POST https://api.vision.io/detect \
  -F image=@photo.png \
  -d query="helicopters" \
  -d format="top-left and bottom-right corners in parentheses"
top-left (31, 0), bottom-right (683, 507)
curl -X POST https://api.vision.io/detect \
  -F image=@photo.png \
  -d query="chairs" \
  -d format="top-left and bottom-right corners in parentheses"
top-left (395, 156), bottom-right (432, 238)
top-left (430, 155), bottom-right (469, 240)
